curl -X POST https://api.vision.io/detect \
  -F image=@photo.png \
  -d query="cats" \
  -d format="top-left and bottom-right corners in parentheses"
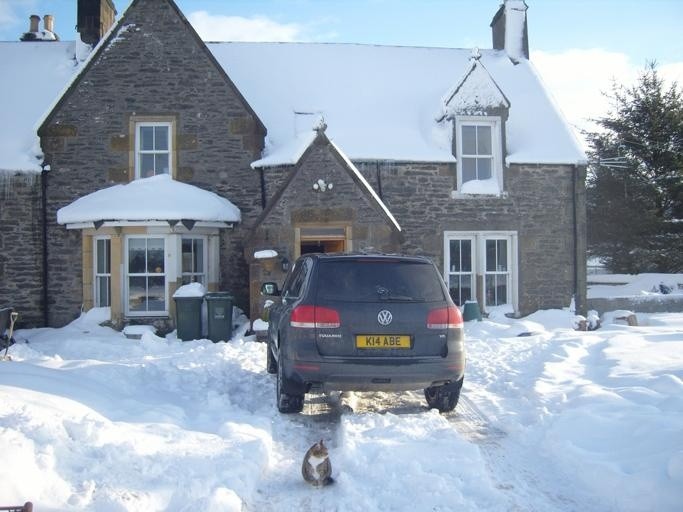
top-left (301, 438), bottom-right (334, 489)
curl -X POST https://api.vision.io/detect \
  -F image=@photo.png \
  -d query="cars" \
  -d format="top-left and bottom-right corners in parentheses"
top-left (261, 251), bottom-right (465, 410)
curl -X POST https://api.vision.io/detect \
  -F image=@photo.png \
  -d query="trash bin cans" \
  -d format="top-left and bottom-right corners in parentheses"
top-left (173, 292), bottom-right (233, 343)
